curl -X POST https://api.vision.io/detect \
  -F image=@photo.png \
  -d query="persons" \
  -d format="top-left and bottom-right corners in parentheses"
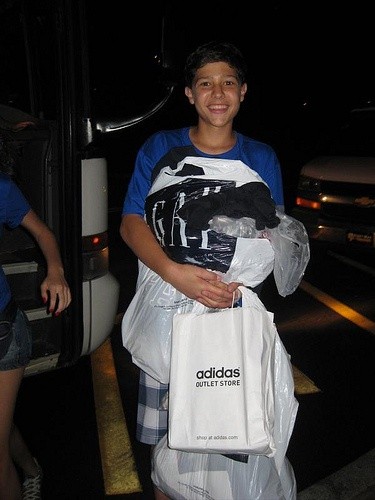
top-left (0, 175), bottom-right (72, 500)
top-left (118, 49), bottom-right (287, 500)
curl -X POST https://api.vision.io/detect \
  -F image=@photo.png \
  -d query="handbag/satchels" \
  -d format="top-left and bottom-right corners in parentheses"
top-left (167, 285), bottom-right (277, 456)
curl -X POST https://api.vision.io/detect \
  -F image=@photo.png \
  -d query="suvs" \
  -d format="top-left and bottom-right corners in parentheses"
top-left (290, 104), bottom-right (374, 274)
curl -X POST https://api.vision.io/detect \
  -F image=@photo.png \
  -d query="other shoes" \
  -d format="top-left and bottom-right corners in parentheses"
top-left (20, 458), bottom-right (42, 500)
